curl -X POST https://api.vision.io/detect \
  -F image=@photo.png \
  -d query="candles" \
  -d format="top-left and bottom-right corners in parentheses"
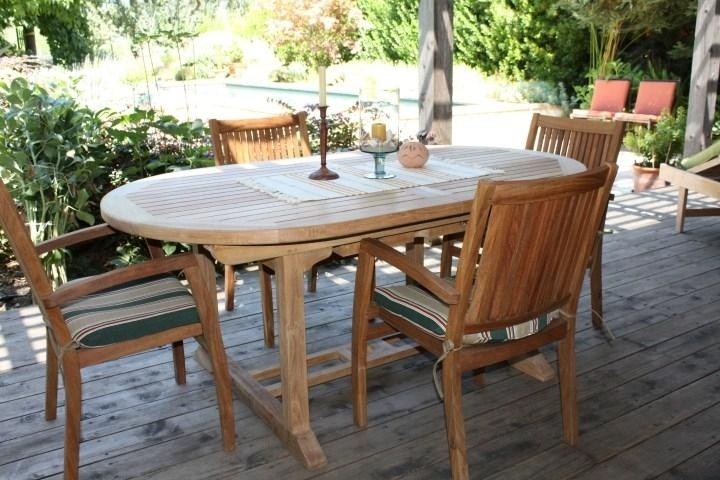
top-left (318, 65), bottom-right (327, 106)
top-left (371, 124), bottom-right (387, 143)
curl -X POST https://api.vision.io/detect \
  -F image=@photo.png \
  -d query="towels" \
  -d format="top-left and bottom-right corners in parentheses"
top-left (238, 153), bottom-right (505, 205)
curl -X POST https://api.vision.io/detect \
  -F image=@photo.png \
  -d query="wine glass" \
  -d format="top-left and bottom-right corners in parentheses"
top-left (356, 86), bottom-right (400, 179)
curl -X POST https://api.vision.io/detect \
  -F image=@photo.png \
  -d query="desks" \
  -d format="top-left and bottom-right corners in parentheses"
top-left (99, 143), bottom-right (590, 470)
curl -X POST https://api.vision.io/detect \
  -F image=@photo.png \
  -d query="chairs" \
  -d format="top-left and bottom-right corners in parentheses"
top-left (616, 80), bottom-right (677, 132)
top-left (208, 111), bottom-right (323, 310)
top-left (439, 114), bottom-right (626, 331)
top-left (659, 141), bottom-right (720, 232)
top-left (572, 79), bottom-right (632, 122)
top-left (0, 177), bottom-right (237, 480)
top-left (349, 162), bottom-right (618, 479)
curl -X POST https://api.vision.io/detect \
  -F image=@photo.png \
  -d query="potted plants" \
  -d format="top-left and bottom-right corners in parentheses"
top-left (622, 106), bottom-right (687, 192)
